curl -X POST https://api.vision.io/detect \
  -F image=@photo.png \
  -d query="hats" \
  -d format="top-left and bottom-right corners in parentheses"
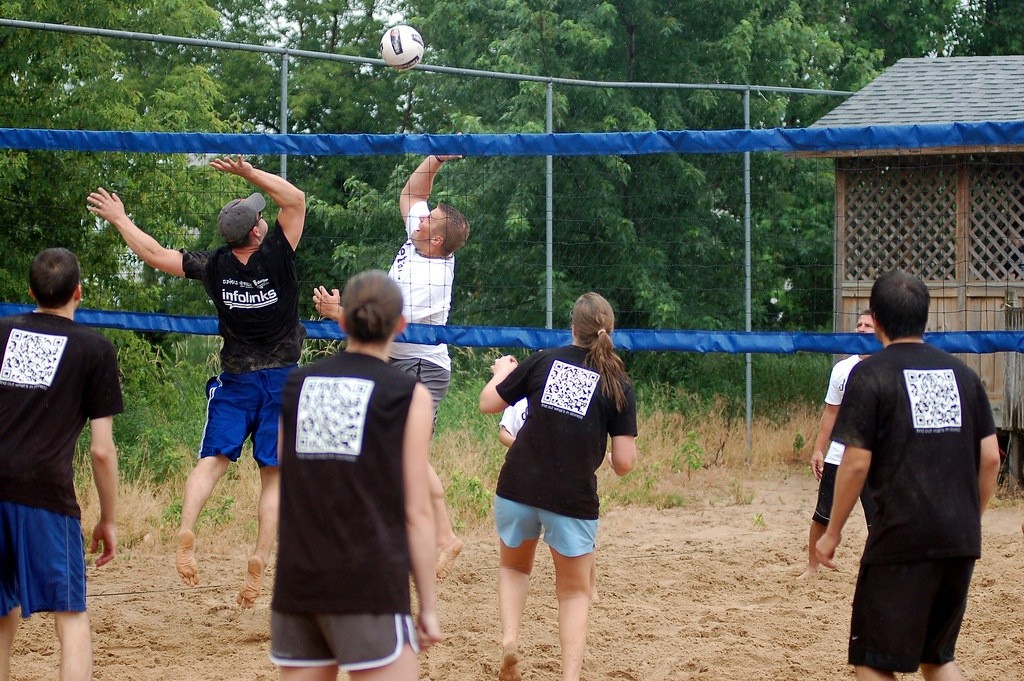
top-left (217, 192), bottom-right (266, 242)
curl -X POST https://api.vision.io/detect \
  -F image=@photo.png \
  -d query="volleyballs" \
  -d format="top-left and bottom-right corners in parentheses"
top-left (380, 24), bottom-right (425, 70)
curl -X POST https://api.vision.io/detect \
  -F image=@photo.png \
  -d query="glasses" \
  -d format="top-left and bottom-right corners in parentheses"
top-left (255, 211), bottom-right (263, 225)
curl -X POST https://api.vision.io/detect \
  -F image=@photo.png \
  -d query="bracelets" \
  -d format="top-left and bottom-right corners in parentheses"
top-left (434, 155), bottom-right (445, 163)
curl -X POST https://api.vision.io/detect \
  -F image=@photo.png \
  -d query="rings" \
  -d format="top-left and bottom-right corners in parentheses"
top-left (97, 202), bottom-right (102, 208)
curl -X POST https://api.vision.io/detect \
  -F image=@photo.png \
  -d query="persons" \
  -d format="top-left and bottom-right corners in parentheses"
top-left (815, 268), bottom-right (1001, 681)
top-left (478, 291), bottom-right (638, 681)
top-left (797, 309), bottom-right (875, 578)
top-left (499, 397), bottom-right (601, 604)
top-left (86, 156), bottom-right (306, 611)
top-left (0, 249), bottom-right (122, 680)
top-left (268, 268), bottom-right (441, 681)
top-left (313, 131), bottom-right (469, 586)
